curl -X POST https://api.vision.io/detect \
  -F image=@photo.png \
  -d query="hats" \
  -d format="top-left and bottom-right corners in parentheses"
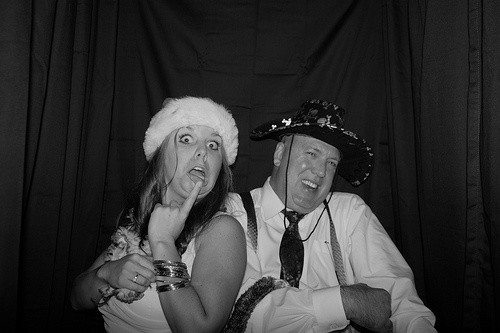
top-left (249, 96), bottom-right (375, 187)
top-left (142, 97), bottom-right (238, 165)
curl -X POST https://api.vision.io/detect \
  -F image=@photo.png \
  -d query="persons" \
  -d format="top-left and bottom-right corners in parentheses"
top-left (226, 98), bottom-right (437, 333)
top-left (73, 95), bottom-right (248, 333)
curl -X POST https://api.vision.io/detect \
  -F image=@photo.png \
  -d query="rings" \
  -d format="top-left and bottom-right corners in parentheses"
top-left (133, 273), bottom-right (140, 283)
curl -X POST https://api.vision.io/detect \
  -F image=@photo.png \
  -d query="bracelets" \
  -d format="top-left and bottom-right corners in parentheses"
top-left (152, 258), bottom-right (191, 293)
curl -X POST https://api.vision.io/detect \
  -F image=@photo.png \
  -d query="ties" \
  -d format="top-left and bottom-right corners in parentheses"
top-left (278, 208), bottom-right (309, 289)
